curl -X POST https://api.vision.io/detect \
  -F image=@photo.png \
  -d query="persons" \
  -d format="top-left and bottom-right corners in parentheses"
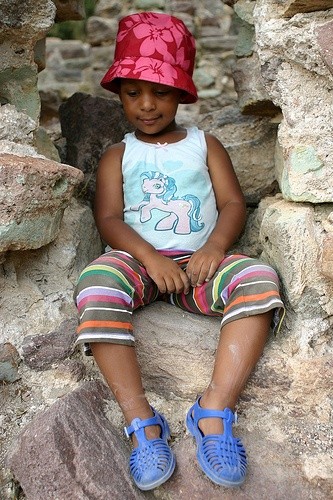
top-left (73, 12), bottom-right (286, 488)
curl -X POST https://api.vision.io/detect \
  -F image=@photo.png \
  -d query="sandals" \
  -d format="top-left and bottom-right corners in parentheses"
top-left (185, 396), bottom-right (247, 487)
top-left (124, 408), bottom-right (176, 490)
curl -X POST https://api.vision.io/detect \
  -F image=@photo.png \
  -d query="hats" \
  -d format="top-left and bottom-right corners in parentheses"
top-left (101, 12), bottom-right (198, 105)
